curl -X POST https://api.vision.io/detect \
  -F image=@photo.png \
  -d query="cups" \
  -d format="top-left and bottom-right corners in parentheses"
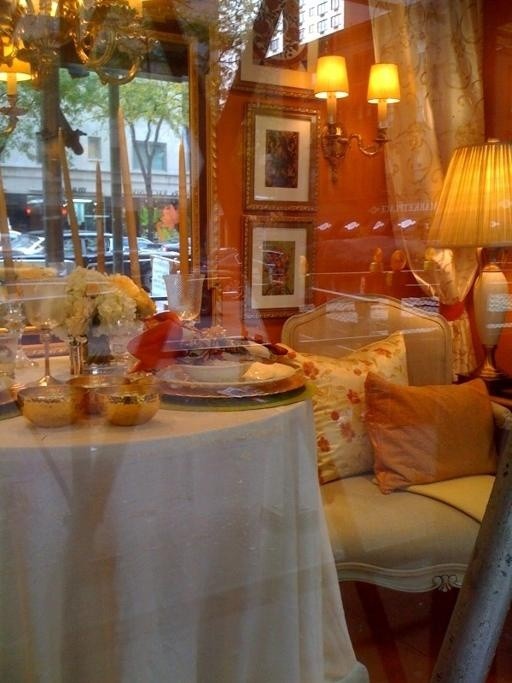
top-left (164, 273), bottom-right (203, 321)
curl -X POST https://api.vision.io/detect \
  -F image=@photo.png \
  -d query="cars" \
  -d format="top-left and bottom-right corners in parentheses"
top-left (0, 228), bottom-right (22, 248)
top-left (0, 228), bottom-right (179, 301)
top-left (160, 235), bottom-right (192, 254)
top-left (119, 234), bottom-right (162, 251)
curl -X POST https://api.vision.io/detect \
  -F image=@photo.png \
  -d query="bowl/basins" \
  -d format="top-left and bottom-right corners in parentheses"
top-left (18, 374), bottom-right (161, 427)
top-left (179, 358), bottom-right (250, 381)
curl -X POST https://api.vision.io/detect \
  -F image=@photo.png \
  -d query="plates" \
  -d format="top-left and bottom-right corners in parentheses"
top-left (137, 361), bottom-right (308, 401)
top-left (1, 387), bottom-right (17, 407)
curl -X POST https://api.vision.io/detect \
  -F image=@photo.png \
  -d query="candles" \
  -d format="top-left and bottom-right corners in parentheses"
top-left (0, 110), bottom-right (187, 310)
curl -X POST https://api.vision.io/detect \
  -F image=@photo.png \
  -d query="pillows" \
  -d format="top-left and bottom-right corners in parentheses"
top-left (278, 329), bottom-right (410, 486)
top-left (366, 373), bottom-right (499, 493)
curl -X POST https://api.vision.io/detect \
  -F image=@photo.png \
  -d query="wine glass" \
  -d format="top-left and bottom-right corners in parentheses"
top-left (0, 285), bottom-right (137, 384)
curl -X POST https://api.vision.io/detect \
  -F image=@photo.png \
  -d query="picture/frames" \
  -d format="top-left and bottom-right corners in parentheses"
top-left (243, 103), bottom-right (317, 214)
top-left (240, 213), bottom-right (314, 321)
top-left (216, 0), bottom-right (332, 100)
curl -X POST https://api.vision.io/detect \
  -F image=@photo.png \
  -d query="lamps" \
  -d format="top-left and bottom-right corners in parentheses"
top-left (422, 138), bottom-right (511, 392)
top-left (313, 55), bottom-right (402, 184)
top-left (0, 1), bottom-right (158, 136)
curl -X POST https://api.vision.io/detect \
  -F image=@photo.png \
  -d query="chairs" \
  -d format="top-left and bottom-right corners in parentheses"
top-left (278, 296), bottom-right (512, 599)
